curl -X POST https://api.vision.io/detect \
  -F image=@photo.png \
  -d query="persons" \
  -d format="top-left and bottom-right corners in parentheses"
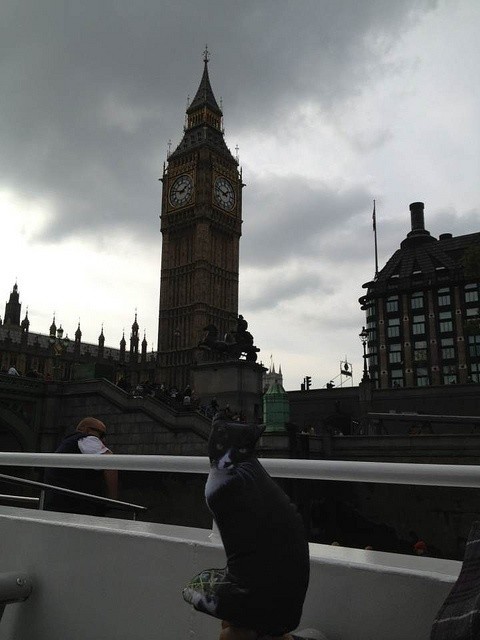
top-left (46, 417), bottom-right (119, 517)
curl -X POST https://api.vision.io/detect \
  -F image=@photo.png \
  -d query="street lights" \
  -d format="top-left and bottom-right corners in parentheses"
top-left (359, 326), bottom-right (370, 376)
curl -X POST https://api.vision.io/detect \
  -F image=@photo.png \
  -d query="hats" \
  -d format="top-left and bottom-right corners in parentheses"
top-left (76, 417), bottom-right (106, 433)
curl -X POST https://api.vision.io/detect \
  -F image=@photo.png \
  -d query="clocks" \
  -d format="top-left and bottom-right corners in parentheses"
top-left (214, 176), bottom-right (237, 211)
top-left (165, 173), bottom-right (193, 208)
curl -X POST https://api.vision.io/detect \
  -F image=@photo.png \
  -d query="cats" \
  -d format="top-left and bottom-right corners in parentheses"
top-left (181, 410), bottom-right (312, 635)
